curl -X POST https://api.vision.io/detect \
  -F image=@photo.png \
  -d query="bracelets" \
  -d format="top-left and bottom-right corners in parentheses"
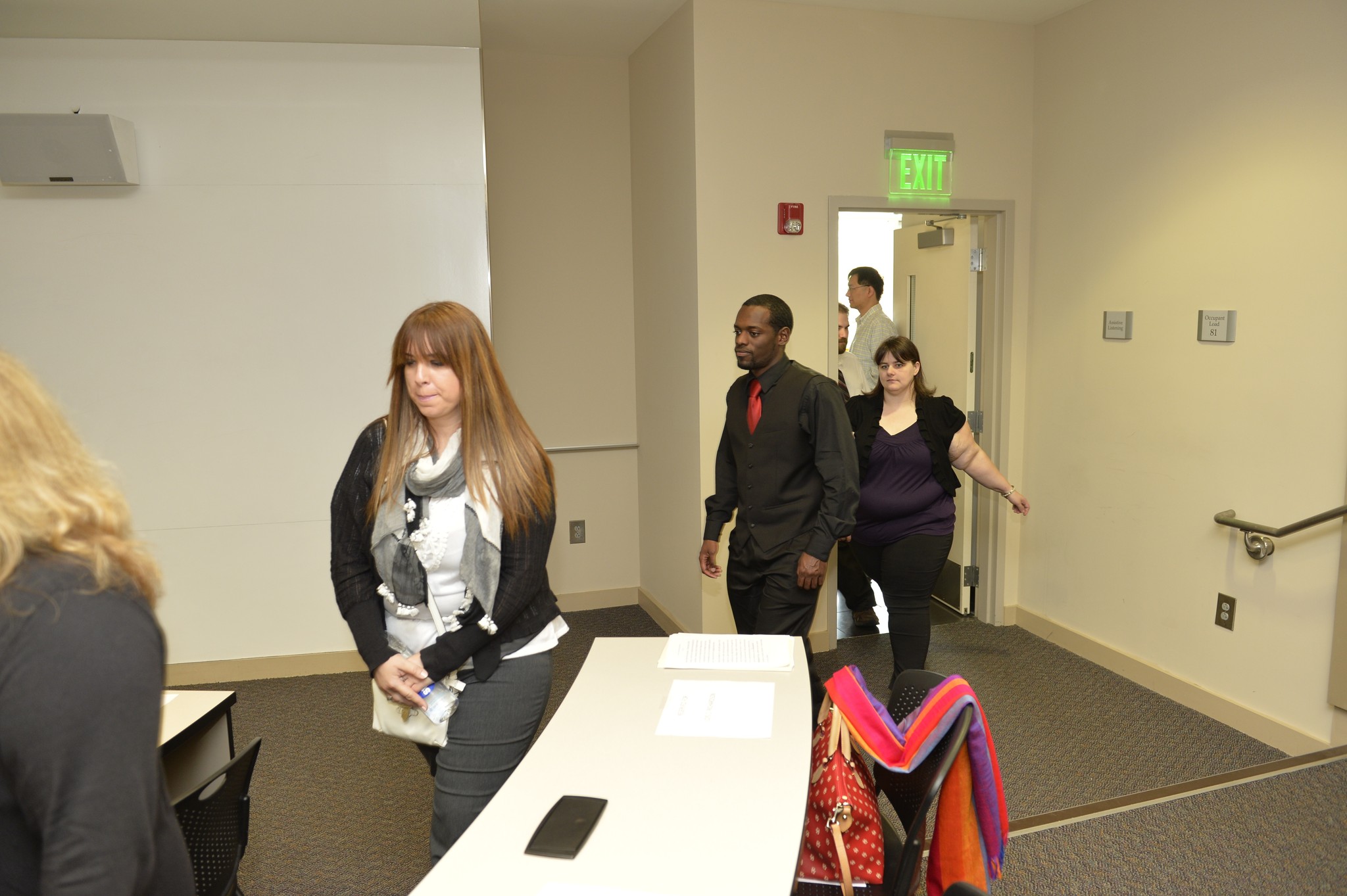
top-left (1001, 485), bottom-right (1015, 497)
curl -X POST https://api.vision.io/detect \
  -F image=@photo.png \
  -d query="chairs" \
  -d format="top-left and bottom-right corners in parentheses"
top-left (795, 669), bottom-right (975, 896)
top-left (170, 736), bottom-right (264, 895)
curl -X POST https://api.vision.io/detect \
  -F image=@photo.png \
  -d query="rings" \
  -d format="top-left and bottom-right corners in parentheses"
top-left (387, 696), bottom-right (392, 702)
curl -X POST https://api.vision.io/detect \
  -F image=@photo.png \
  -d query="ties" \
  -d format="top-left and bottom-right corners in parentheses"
top-left (745, 380), bottom-right (762, 436)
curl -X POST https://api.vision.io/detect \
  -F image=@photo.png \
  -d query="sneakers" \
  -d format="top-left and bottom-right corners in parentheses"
top-left (851, 606), bottom-right (880, 626)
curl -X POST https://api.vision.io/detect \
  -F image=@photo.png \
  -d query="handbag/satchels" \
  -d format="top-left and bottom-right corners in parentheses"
top-left (371, 674), bottom-right (467, 748)
top-left (792, 690), bottom-right (884, 896)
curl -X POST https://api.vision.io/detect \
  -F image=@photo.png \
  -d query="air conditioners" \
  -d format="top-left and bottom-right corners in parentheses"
top-left (0, 113), bottom-right (140, 187)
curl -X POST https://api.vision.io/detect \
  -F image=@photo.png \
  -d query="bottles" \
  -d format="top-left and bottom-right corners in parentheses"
top-left (383, 630), bottom-right (459, 725)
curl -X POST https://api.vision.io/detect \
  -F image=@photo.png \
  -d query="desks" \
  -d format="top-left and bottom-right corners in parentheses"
top-left (156, 688), bottom-right (237, 809)
top-left (405, 632), bottom-right (814, 896)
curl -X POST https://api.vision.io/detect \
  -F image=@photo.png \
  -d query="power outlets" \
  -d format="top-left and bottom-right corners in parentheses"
top-left (1215, 592), bottom-right (1237, 632)
top-left (569, 520), bottom-right (585, 544)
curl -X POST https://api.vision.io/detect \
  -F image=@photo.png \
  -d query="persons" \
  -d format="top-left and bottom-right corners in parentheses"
top-left (329, 300), bottom-right (569, 875)
top-left (844, 334), bottom-right (1031, 694)
top-left (0, 344), bottom-right (197, 893)
top-left (838, 302), bottom-right (874, 403)
top-left (845, 266), bottom-right (900, 393)
top-left (700, 295), bottom-right (862, 723)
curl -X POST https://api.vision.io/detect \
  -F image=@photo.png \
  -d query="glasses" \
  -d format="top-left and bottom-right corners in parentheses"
top-left (847, 285), bottom-right (870, 293)
